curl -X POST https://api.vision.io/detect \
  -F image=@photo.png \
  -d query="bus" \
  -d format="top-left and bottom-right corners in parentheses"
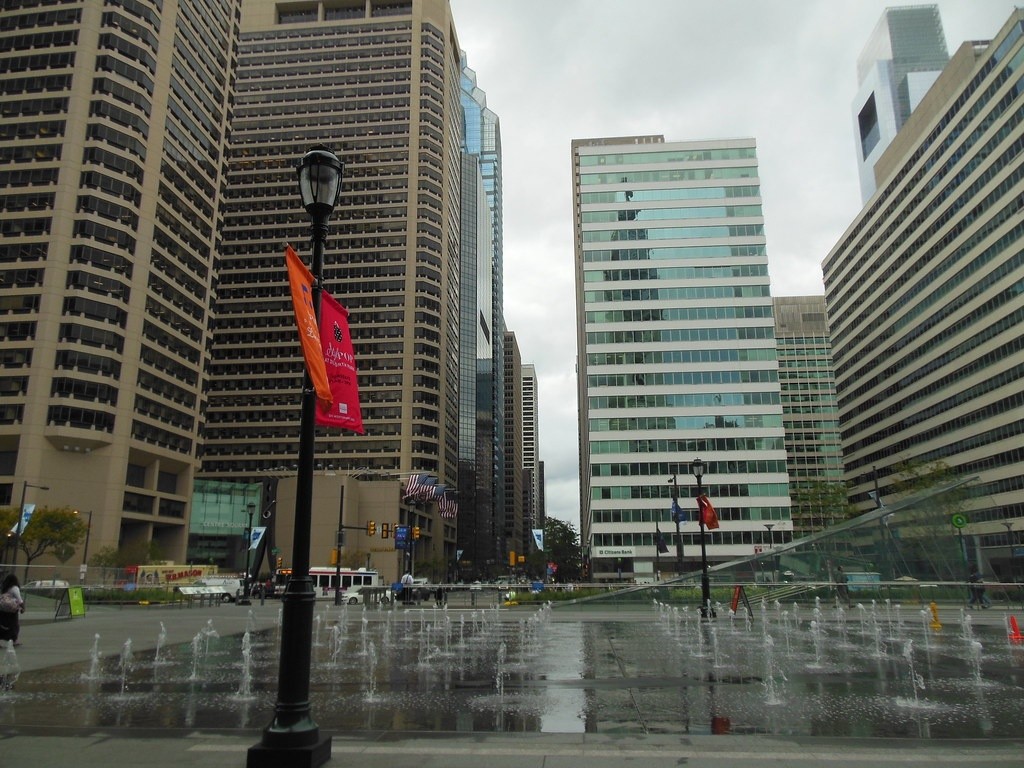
top-left (124, 563), bottom-right (218, 591)
top-left (274, 566), bottom-right (379, 599)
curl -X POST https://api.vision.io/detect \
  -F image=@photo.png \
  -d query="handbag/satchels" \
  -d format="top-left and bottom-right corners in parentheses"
top-left (0, 591), bottom-right (19, 613)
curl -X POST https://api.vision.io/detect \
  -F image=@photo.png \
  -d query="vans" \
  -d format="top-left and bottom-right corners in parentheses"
top-left (185, 576), bottom-right (245, 603)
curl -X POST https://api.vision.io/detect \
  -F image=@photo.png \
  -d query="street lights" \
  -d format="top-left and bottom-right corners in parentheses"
top-left (245, 146), bottom-right (344, 768)
top-left (690, 457), bottom-right (716, 618)
top-left (666, 471), bottom-right (686, 585)
top-left (764, 523), bottom-right (776, 589)
top-left (72, 509), bottom-right (93, 585)
top-left (11, 480), bottom-right (50, 578)
top-left (1001, 523), bottom-right (1016, 584)
top-left (240, 503), bottom-right (255, 606)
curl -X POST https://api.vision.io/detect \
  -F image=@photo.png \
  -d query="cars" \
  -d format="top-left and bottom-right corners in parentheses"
top-left (20, 580), bottom-right (70, 589)
top-left (342, 584), bottom-right (392, 605)
top-left (469, 576), bottom-right (521, 592)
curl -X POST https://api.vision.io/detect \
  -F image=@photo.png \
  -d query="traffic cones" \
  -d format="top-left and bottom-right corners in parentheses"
top-left (1008, 615), bottom-right (1024, 639)
top-left (928, 602), bottom-right (943, 627)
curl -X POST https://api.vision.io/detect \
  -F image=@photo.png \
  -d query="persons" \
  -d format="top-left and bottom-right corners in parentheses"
top-left (0, 574), bottom-right (25, 647)
top-left (253, 578), bottom-right (271, 598)
top-left (966, 565), bottom-right (988, 609)
top-left (833, 566), bottom-right (855, 608)
top-left (434, 583), bottom-right (443, 605)
top-left (401, 570), bottom-right (414, 602)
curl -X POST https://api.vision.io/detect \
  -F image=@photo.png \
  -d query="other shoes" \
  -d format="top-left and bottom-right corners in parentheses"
top-left (13, 640), bottom-right (23, 645)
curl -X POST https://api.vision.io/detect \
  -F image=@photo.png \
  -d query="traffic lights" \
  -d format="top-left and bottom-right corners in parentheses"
top-left (369, 520), bottom-right (376, 534)
top-left (414, 525), bottom-right (420, 540)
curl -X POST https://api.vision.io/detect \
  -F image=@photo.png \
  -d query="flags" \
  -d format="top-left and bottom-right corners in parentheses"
top-left (402, 474), bottom-right (459, 518)
